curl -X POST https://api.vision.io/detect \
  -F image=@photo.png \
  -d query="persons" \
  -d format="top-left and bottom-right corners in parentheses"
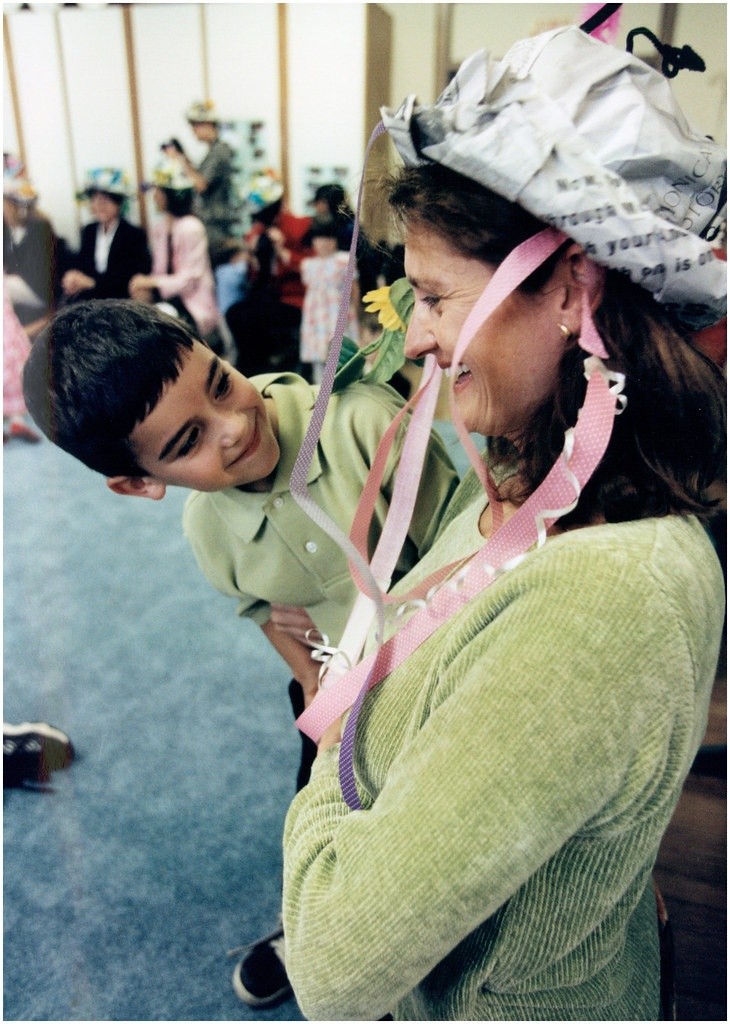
top-left (3, 100), bottom-right (404, 446)
top-left (21, 295), bottom-right (459, 1007)
top-left (279, 24), bottom-right (728, 1021)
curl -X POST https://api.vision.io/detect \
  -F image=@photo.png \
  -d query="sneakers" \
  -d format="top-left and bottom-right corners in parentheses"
top-left (3, 721), bottom-right (74, 794)
top-left (227, 926), bottom-right (291, 1006)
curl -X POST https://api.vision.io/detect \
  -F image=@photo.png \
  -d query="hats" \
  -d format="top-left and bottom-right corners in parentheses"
top-left (151, 158), bottom-right (194, 189)
top-left (378, 25), bottom-right (727, 311)
top-left (185, 100), bottom-right (220, 122)
top-left (76, 167), bottom-right (130, 200)
top-left (3, 154), bottom-right (38, 202)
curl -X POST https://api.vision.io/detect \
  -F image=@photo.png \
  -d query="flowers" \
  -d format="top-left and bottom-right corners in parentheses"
top-left (308, 276), bottom-right (416, 409)
